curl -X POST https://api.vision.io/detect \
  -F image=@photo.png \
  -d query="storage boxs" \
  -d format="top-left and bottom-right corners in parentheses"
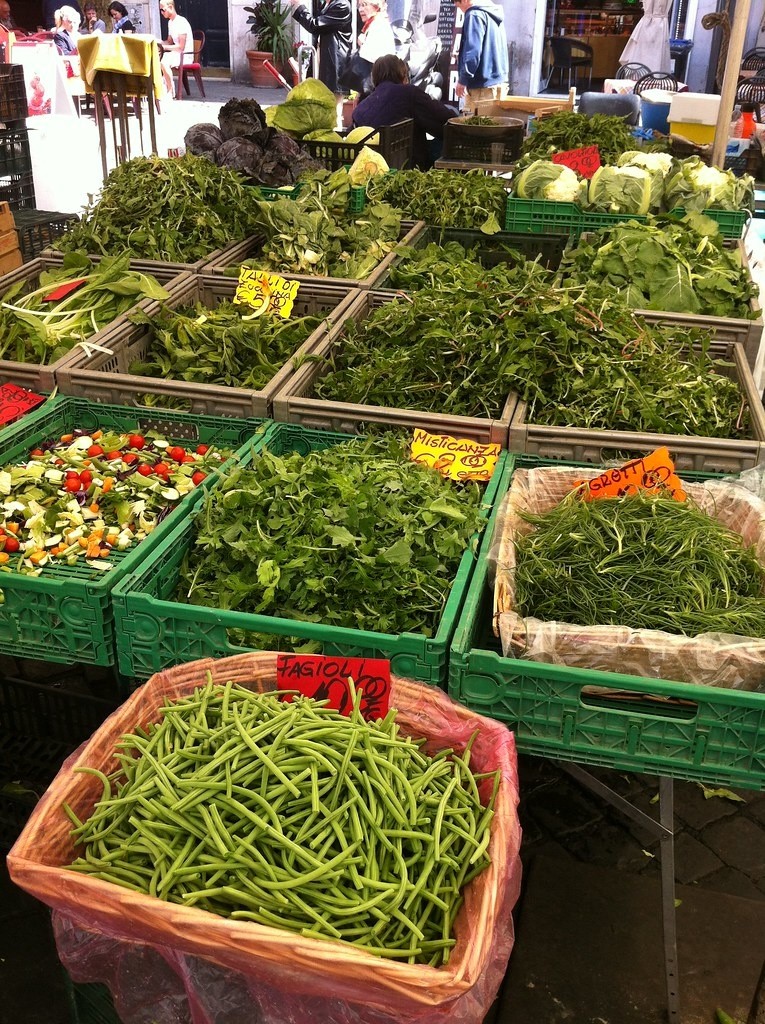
top-left (0, 64), bottom-right (29, 121)
top-left (0, 120), bottom-right (33, 190)
top-left (442, 122), bottom-right (523, 163)
top-left (291, 117), bottom-right (414, 174)
top-left (669, 122), bottom-right (717, 145)
top-left (667, 91), bottom-right (722, 125)
top-left (0, 177), bottom-right (765, 792)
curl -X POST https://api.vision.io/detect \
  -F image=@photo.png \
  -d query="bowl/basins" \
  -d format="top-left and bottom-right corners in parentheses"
top-left (447, 116), bottom-right (523, 137)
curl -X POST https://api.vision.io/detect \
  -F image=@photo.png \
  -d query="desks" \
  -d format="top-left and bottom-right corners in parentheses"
top-left (601, 79), bottom-right (689, 95)
top-left (543, 34), bottom-right (630, 79)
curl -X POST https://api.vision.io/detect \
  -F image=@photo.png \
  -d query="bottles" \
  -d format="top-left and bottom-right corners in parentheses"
top-left (726, 105), bottom-right (744, 154)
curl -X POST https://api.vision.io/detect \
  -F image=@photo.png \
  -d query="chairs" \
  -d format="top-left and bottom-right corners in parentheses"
top-left (733, 46), bottom-right (765, 123)
top-left (171, 30), bottom-right (206, 100)
top-left (577, 92), bottom-right (641, 126)
top-left (633, 71), bottom-right (678, 98)
top-left (0, 23), bottom-right (164, 121)
top-left (616, 62), bottom-right (654, 81)
top-left (545, 36), bottom-right (593, 91)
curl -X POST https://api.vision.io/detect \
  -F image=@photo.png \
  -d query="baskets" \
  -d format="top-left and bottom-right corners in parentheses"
top-left (0, 118), bottom-right (765, 791)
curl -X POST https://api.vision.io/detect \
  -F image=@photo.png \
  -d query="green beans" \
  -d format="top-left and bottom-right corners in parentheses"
top-left (64, 676), bottom-right (500, 968)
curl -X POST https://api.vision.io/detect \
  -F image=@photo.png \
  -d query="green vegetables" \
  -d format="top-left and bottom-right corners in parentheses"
top-left (517, 110), bottom-right (665, 167)
top-left (532, 212), bottom-right (764, 439)
top-left (0, 429), bottom-right (497, 655)
top-left (0, 153), bottom-right (576, 462)
top-left (510, 481), bottom-right (765, 640)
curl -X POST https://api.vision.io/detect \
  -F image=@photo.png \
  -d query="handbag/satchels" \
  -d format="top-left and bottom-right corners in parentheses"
top-left (338, 58), bottom-right (373, 92)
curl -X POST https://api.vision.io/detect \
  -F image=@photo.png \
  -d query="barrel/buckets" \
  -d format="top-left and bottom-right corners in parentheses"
top-left (641, 99), bottom-right (670, 135)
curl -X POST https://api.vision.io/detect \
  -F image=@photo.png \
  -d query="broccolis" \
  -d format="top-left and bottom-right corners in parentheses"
top-left (514, 150), bottom-right (755, 213)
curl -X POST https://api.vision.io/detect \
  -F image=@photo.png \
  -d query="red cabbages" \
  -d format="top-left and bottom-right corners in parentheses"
top-left (184, 99), bottom-right (324, 188)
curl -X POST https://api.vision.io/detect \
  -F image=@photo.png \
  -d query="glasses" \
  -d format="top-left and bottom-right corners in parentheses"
top-left (159, 6), bottom-right (169, 12)
top-left (357, 2), bottom-right (369, 9)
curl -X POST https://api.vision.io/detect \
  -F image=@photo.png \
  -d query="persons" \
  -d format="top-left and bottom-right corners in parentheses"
top-left (290, 0), bottom-right (395, 94)
top-left (0, 0), bottom-right (13, 49)
top-left (452, 0), bottom-right (509, 109)
top-left (159, 0), bottom-right (193, 98)
top-left (81, 4), bottom-right (105, 35)
top-left (108, 1), bottom-right (133, 34)
top-left (352, 54), bottom-right (457, 171)
top-left (54, 6), bottom-right (109, 116)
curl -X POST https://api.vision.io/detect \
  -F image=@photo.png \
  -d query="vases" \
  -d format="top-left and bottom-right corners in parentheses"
top-left (291, 68), bottom-right (298, 88)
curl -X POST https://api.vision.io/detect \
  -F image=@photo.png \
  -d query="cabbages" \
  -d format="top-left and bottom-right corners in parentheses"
top-left (262, 77), bottom-right (379, 145)
top-left (348, 145), bottom-right (390, 182)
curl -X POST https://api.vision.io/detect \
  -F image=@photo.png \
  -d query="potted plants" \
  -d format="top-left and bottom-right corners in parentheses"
top-left (243, 0), bottom-right (300, 89)
top-left (342, 89), bottom-right (358, 126)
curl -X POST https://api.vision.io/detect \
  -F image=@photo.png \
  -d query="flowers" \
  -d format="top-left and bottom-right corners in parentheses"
top-left (292, 41), bottom-right (310, 65)
